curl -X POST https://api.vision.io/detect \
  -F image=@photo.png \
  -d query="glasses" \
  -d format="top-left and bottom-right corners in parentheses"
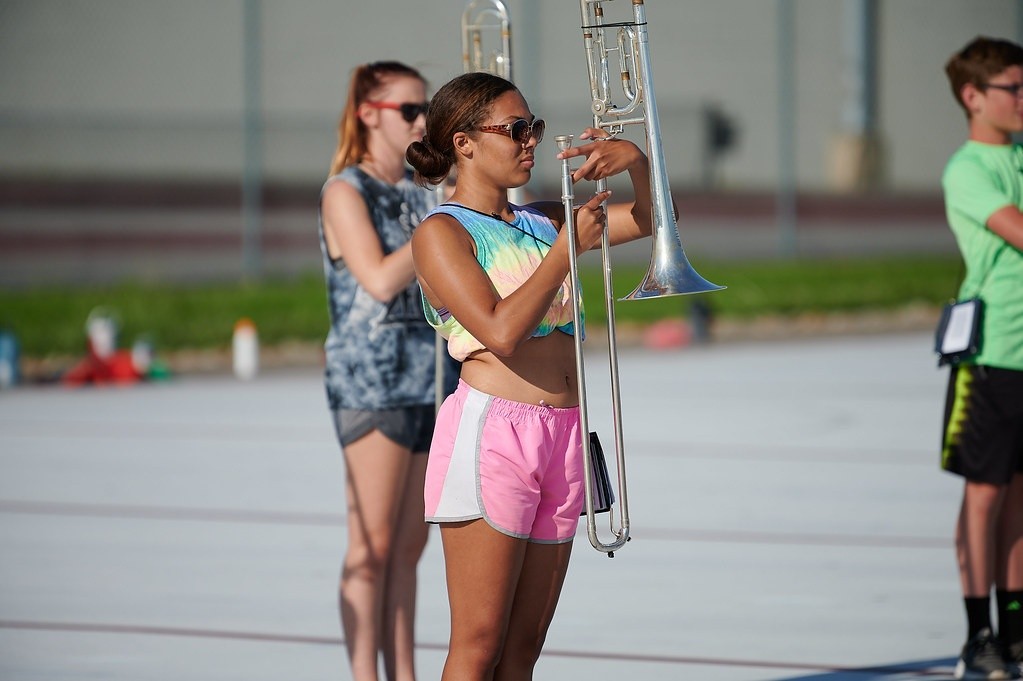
top-left (356, 101), bottom-right (429, 122)
top-left (466, 120), bottom-right (545, 144)
top-left (980, 82), bottom-right (1023, 94)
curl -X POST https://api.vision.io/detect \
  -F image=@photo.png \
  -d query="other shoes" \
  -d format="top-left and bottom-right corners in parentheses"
top-left (954, 641), bottom-right (1012, 681)
top-left (996, 633), bottom-right (1023, 676)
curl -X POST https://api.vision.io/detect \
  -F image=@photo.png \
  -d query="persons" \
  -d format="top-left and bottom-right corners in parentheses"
top-left (935, 33), bottom-right (1023, 681)
top-left (317, 62), bottom-right (454, 681)
top-left (404, 72), bottom-right (680, 681)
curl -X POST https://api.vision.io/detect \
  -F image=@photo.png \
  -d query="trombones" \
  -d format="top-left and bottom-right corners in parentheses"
top-left (464, 2), bottom-right (527, 210)
top-left (558, 0), bottom-right (725, 556)
